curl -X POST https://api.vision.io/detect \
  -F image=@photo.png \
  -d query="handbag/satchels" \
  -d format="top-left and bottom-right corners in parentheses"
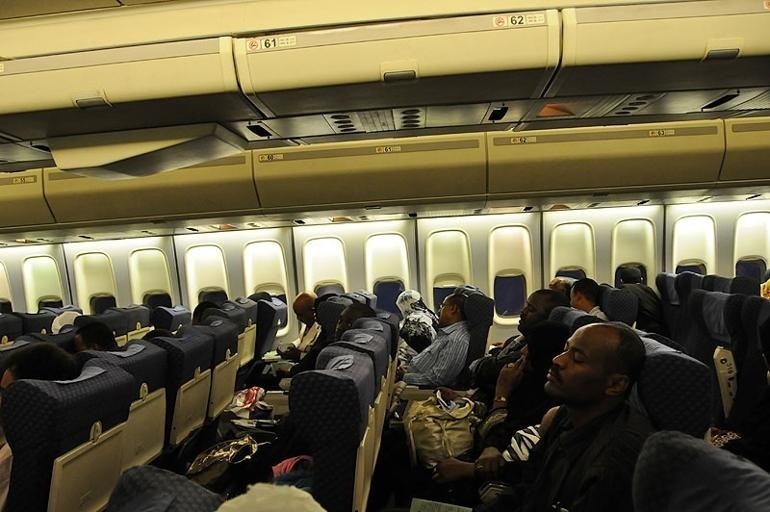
top-left (401, 389), bottom-right (477, 479)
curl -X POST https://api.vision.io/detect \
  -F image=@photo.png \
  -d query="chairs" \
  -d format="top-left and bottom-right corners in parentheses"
top-left (1, 292), bottom-right (288, 511)
top-left (624, 328), bottom-right (719, 440)
top-left (596, 282), bottom-right (639, 328)
top-left (277, 329), bottom-right (390, 476)
top-left (348, 308), bottom-right (400, 411)
top-left (655, 273), bottom-right (770, 469)
top-left (389, 285), bottom-right (495, 429)
top-left (633, 430), bottom-right (769, 511)
top-left (227, 346), bottom-right (376, 510)
top-left (508, 306), bottom-right (602, 427)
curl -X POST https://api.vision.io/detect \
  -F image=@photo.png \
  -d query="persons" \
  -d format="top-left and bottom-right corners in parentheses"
top-left (0, 342), bottom-right (80, 509)
top-left (72, 322), bottom-right (122, 357)
top-left (238, 294), bottom-right (375, 391)
